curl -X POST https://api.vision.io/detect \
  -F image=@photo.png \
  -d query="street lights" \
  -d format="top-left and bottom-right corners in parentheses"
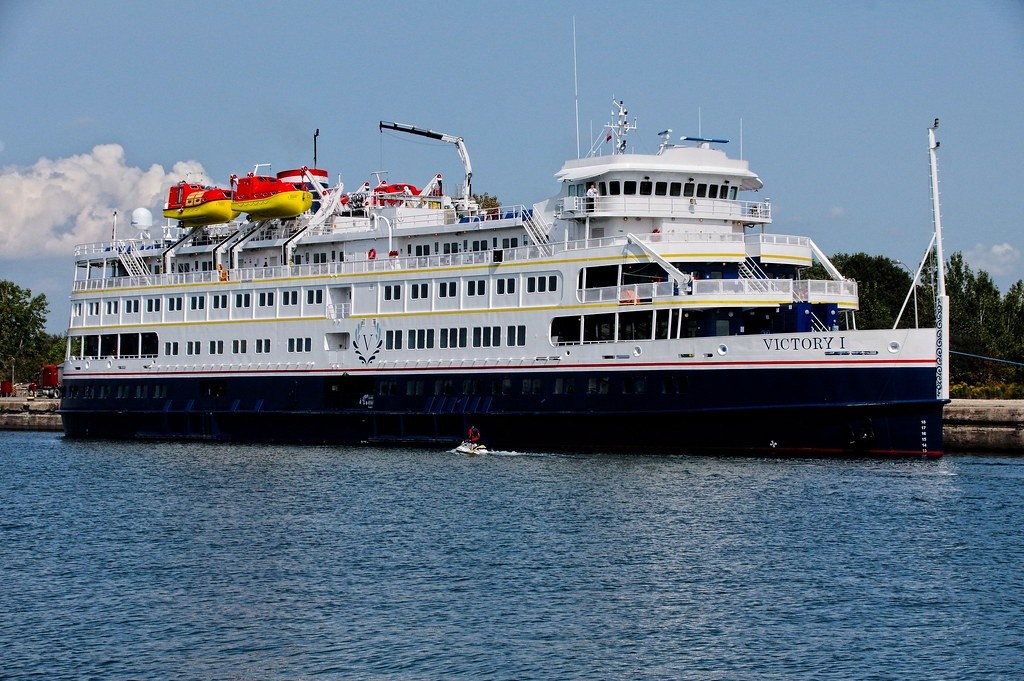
top-left (890, 259), bottom-right (919, 329)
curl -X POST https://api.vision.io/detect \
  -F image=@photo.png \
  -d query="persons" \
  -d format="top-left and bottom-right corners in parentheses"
top-left (465, 426), bottom-right (480, 442)
top-left (289, 260), bottom-right (296, 276)
top-left (586, 185), bottom-right (598, 209)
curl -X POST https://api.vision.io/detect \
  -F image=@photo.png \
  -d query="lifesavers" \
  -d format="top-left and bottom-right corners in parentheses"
top-left (651, 228), bottom-right (661, 242)
top-left (368, 248), bottom-right (377, 259)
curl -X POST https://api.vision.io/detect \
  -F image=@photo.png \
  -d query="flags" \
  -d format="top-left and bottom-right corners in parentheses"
top-left (607, 129), bottom-right (612, 142)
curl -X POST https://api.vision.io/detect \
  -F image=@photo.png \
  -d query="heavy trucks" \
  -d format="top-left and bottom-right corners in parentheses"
top-left (27, 364), bottom-right (65, 398)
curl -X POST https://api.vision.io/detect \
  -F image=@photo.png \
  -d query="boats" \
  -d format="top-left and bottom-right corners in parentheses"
top-left (161, 180), bottom-right (242, 229)
top-left (232, 175), bottom-right (314, 222)
top-left (58, 99), bottom-right (950, 459)
top-left (369, 183), bottom-right (453, 217)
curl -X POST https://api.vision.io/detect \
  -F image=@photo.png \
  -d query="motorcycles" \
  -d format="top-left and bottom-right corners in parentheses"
top-left (453, 438), bottom-right (490, 457)
